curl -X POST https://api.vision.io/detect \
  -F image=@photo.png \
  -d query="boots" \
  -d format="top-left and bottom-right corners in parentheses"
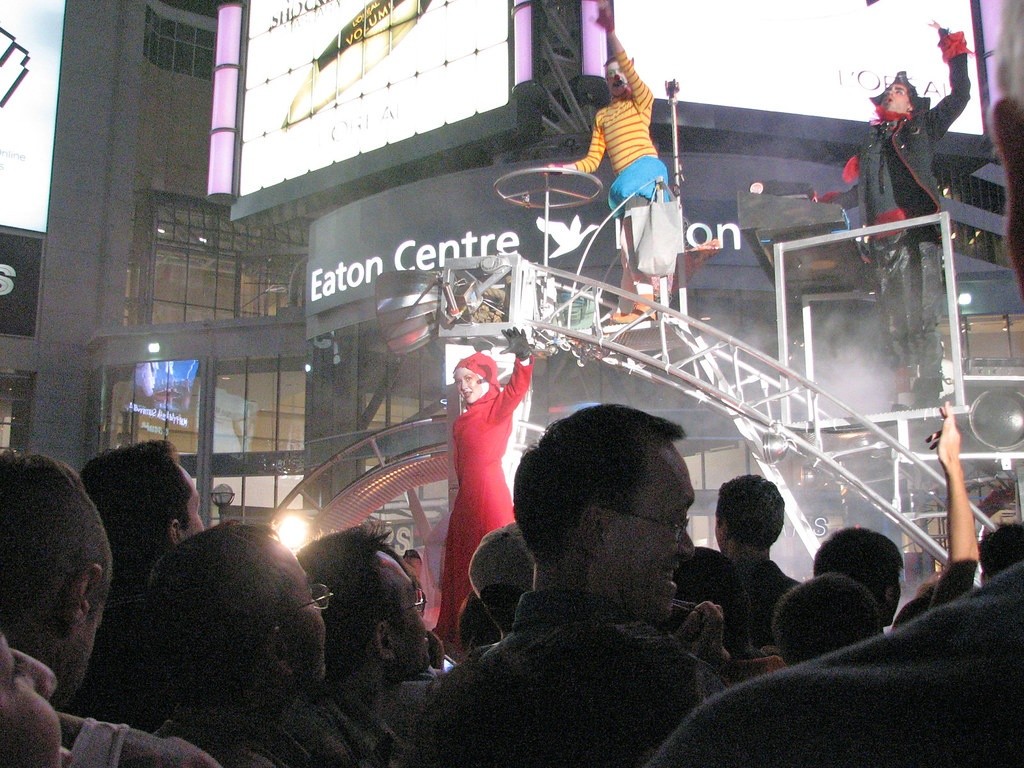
top-left (610, 294), bottom-right (656, 323)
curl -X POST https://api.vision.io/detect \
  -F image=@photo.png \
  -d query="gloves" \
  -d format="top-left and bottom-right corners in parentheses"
top-left (500, 327), bottom-right (530, 361)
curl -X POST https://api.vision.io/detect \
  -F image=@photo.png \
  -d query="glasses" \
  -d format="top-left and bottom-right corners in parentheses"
top-left (275, 583), bottom-right (333, 626)
top-left (385, 588), bottom-right (426, 620)
top-left (600, 506), bottom-right (689, 542)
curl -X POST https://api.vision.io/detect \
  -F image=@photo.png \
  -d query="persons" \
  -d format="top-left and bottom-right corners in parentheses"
top-left (540, 0), bottom-right (669, 326)
top-left (0, 399), bottom-right (1024, 768)
top-left (430, 327), bottom-right (536, 664)
top-left (645, 0), bottom-right (1024, 768)
top-left (810, 19), bottom-right (972, 413)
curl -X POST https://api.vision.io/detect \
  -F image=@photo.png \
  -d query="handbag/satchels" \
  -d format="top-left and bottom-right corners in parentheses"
top-left (629, 182), bottom-right (677, 278)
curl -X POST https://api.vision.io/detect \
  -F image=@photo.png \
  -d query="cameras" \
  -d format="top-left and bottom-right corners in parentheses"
top-left (659, 600), bottom-right (702, 641)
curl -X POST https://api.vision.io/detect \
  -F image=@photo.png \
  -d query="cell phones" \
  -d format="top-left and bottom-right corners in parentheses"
top-left (443, 655), bottom-right (457, 672)
